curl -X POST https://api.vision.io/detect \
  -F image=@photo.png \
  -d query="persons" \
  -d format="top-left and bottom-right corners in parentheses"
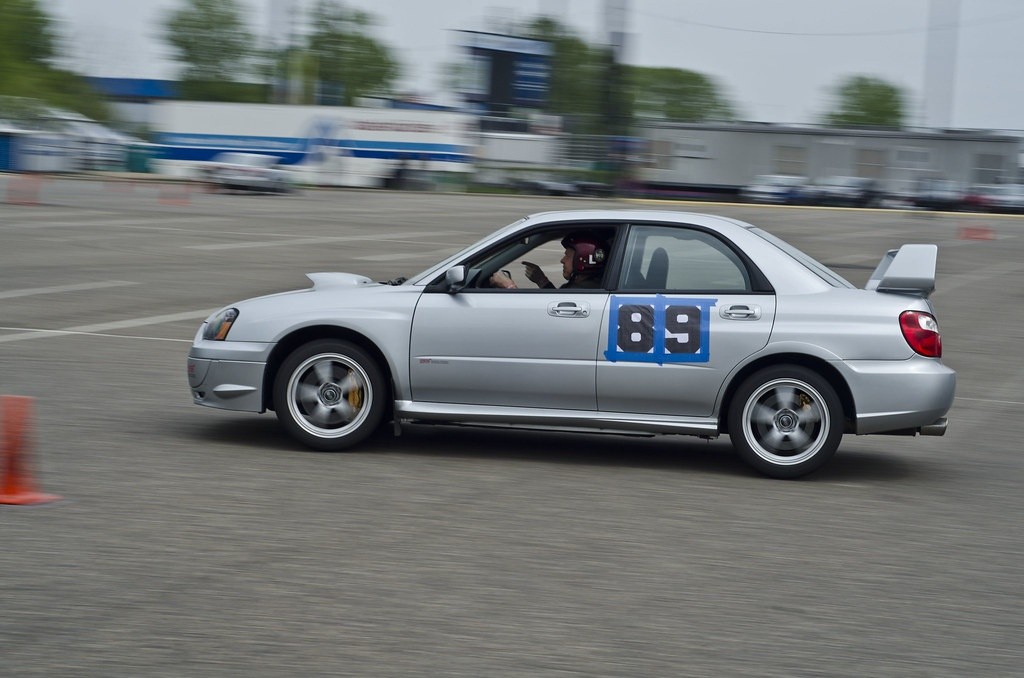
top-left (493, 227), bottom-right (611, 289)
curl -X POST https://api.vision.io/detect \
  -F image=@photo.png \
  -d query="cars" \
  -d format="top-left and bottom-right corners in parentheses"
top-left (188, 209), bottom-right (957, 480)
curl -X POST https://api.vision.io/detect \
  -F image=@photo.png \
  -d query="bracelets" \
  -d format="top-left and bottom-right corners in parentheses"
top-left (506, 284), bottom-right (517, 289)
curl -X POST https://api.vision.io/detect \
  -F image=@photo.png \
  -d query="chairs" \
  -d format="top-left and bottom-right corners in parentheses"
top-left (646, 247), bottom-right (670, 288)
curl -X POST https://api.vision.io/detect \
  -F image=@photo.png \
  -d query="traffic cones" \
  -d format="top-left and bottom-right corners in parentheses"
top-left (0, 395), bottom-right (62, 505)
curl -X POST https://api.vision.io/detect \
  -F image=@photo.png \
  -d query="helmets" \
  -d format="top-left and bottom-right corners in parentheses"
top-left (561, 230), bottom-right (610, 276)
